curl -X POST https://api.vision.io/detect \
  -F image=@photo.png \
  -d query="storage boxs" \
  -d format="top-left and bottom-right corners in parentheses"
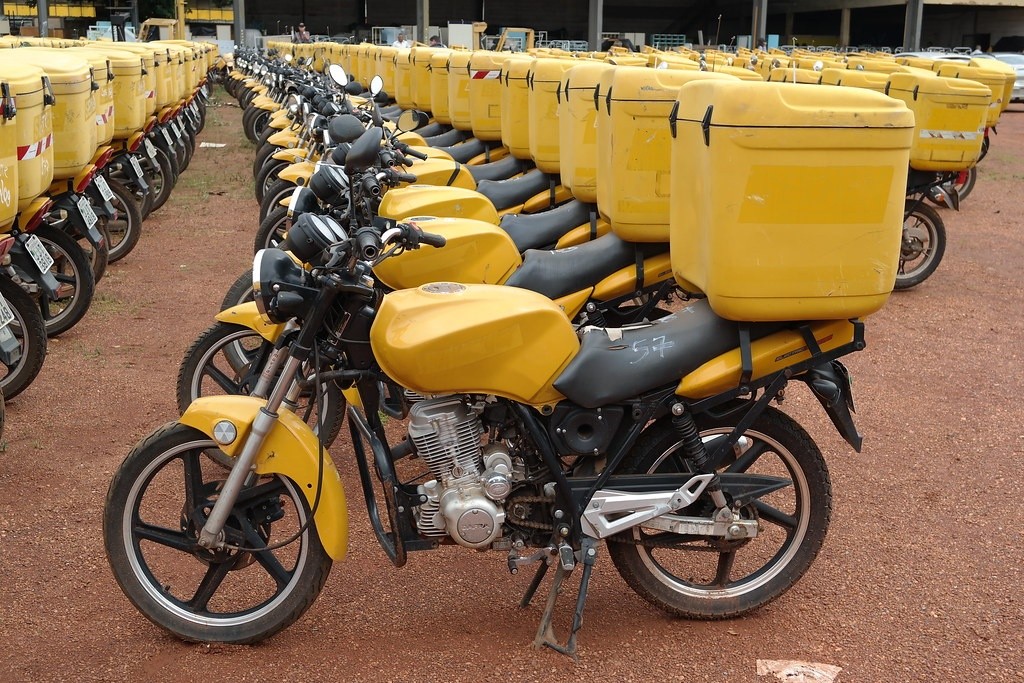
top-left (525, 58), bottom-right (615, 174)
top-left (670, 79), bottom-right (916, 322)
top-left (0, 64), bottom-right (55, 212)
top-left (446, 51), bottom-right (476, 130)
top-left (266, 41), bottom-right (377, 89)
top-left (499, 54), bottom-right (553, 160)
top-left (110, 42), bottom-right (173, 112)
top-left (968, 58), bottom-right (1016, 112)
top-left (94, 47), bottom-right (149, 140)
top-left (931, 61), bottom-right (968, 73)
top-left (594, 66), bottom-right (740, 241)
top-left (885, 72), bottom-right (992, 172)
top-left (392, 47), bottom-right (412, 110)
top-left (818, 68), bottom-right (891, 94)
top-left (466, 50), bottom-right (534, 141)
top-left (374, 46), bottom-right (406, 98)
top-left (557, 61), bottom-right (616, 202)
top-left (48, 47), bottom-right (115, 148)
top-left (0, 48), bottom-right (99, 178)
top-left (525, 46), bottom-right (935, 81)
top-left (937, 64), bottom-right (1007, 127)
top-left (0, 37), bottom-right (218, 105)
top-left (0, 78), bottom-right (18, 234)
top-left (766, 68), bottom-right (822, 85)
top-left (84, 44), bottom-right (160, 120)
top-left (365, 45), bottom-right (389, 89)
top-left (426, 52), bottom-right (451, 124)
top-left (408, 47), bottom-right (456, 112)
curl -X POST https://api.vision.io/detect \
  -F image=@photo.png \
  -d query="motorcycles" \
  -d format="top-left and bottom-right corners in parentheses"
top-left (0, 36), bottom-right (1012, 648)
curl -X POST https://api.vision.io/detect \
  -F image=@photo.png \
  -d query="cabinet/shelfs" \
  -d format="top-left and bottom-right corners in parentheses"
top-left (621, 33), bottom-right (634, 47)
top-left (601, 32), bottom-right (620, 46)
top-left (651, 33), bottom-right (686, 52)
top-left (634, 33), bottom-right (646, 49)
top-left (484, 35), bottom-right (522, 53)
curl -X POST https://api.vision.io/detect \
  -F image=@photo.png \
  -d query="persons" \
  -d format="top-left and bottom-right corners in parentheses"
top-left (292, 22), bottom-right (310, 43)
top-left (758, 38), bottom-right (767, 52)
top-left (429, 35), bottom-right (448, 49)
top-left (391, 33), bottom-right (410, 49)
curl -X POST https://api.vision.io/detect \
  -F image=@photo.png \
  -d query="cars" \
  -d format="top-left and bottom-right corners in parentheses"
top-left (890, 51), bottom-right (1024, 105)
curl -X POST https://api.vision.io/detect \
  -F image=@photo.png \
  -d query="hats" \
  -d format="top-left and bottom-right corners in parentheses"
top-left (758, 38), bottom-right (766, 42)
top-left (299, 23), bottom-right (304, 27)
